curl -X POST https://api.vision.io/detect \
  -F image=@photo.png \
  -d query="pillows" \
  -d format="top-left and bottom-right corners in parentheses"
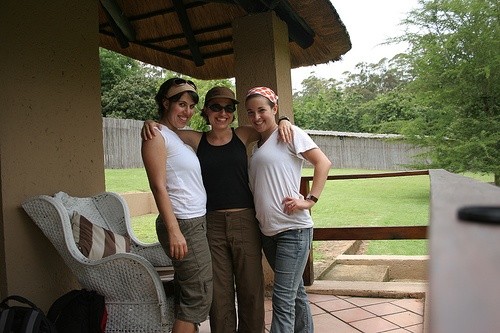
top-left (71, 211), bottom-right (130, 260)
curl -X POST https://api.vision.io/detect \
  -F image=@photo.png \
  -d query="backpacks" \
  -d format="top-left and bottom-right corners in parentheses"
top-left (46, 287), bottom-right (107, 333)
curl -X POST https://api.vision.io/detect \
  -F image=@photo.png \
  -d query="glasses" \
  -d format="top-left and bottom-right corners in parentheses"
top-left (171, 78), bottom-right (197, 90)
top-left (206, 103), bottom-right (236, 113)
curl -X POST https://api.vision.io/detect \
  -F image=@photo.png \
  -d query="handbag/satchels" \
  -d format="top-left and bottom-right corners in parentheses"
top-left (0, 295), bottom-right (54, 333)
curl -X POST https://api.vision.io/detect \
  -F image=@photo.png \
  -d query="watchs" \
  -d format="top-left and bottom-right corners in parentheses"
top-left (306, 194), bottom-right (318, 203)
top-left (278, 115), bottom-right (290, 124)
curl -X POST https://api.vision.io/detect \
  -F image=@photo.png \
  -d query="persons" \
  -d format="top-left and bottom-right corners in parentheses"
top-left (141, 77), bottom-right (213, 333)
top-left (244, 87), bottom-right (332, 333)
top-left (141, 87), bottom-right (295, 333)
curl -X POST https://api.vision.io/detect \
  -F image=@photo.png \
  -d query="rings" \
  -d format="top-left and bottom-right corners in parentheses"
top-left (291, 204), bottom-right (293, 206)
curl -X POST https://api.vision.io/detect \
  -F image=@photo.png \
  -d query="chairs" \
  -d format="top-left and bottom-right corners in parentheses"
top-left (21, 192), bottom-right (178, 333)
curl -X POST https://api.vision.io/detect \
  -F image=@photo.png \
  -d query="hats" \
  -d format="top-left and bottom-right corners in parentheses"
top-left (205, 86), bottom-right (240, 104)
